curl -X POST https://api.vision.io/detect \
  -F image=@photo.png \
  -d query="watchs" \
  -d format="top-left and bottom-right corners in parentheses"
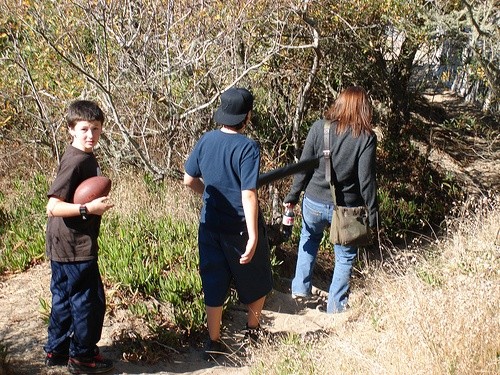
top-left (79, 204), bottom-right (87, 217)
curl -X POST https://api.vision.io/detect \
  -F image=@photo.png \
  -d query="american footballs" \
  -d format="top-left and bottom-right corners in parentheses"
top-left (74, 176), bottom-right (112, 205)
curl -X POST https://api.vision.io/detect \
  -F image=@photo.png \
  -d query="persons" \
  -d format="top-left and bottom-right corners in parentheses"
top-left (283, 87), bottom-right (379, 314)
top-left (184, 88), bottom-right (271, 352)
top-left (46, 100), bottom-right (115, 375)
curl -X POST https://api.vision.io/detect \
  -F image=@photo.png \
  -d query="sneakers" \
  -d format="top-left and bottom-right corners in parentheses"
top-left (67, 347), bottom-right (112, 374)
top-left (46, 351), bottom-right (69, 366)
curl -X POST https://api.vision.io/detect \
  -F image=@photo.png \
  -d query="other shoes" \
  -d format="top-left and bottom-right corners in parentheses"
top-left (204, 340), bottom-right (225, 360)
top-left (245, 325), bottom-right (274, 344)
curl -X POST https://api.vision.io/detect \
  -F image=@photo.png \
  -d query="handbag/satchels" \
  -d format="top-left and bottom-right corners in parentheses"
top-left (328, 206), bottom-right (373, 249)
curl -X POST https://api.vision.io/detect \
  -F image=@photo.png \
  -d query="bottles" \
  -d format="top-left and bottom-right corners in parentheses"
top-left (281, 206), bottom-right (294, 237)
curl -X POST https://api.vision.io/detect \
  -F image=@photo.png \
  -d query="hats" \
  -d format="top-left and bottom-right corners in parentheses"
top-left (213, 89), bottom-right (252, 125)
